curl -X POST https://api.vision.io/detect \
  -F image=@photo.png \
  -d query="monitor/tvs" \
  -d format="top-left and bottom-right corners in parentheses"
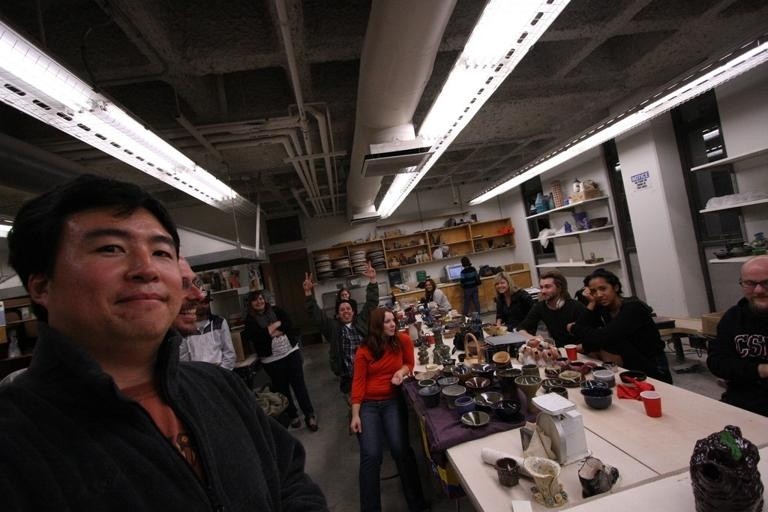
top-left (445, 263), bottom-right (464, 281)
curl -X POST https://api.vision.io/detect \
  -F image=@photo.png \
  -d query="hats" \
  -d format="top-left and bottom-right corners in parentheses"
top-left (262, 334), bottom-right (299, 364)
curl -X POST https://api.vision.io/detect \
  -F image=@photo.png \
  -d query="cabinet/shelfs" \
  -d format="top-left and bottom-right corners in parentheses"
top-left (516, 147), bottom-right (637, 307)
top-left (680, 147), bottom-right (767, 315)
top-left (310, 218), bottom-right (533, 325)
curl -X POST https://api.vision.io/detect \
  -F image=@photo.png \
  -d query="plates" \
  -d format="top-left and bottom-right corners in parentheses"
top-left (314, 248), bottom-right (387, 278)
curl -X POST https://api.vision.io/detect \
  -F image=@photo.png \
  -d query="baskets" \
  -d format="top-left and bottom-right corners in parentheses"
top-left (464, 332), bottom-right (483, 367)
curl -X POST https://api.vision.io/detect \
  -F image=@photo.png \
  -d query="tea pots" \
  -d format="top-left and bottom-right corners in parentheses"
top-left (713, 249), bottom-right (730, 259)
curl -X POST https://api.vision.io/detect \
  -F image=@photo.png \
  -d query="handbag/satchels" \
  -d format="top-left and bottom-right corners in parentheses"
top-left (454, 324), bottom-right (483, 350)
top-left (254, 383), bottom-right (288, 418)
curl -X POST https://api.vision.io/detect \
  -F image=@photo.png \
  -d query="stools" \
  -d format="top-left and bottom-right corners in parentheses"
top-left (653, 315), bottom-right (684, 363)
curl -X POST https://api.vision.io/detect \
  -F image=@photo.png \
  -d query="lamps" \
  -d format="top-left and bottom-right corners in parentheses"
top-left (378, 0), bottom-right (571, 216)
top-left (0, 20), bottom-right (258, 217)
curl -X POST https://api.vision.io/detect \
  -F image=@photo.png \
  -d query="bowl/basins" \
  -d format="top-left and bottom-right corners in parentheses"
top-left (484, 324), bottom-right (509, 336)
top-left (414, 351), bottom-right (521, 428)
top-left (541, 360), bottom-right (648, 394)
top-left (590, 218), bottom-right (608, 227)
top-left (582, 387), bottom-right (614, 410)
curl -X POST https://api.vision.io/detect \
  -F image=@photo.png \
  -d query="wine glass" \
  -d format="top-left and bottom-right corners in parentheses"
top-left (515, 375), bottom-right (542, 414)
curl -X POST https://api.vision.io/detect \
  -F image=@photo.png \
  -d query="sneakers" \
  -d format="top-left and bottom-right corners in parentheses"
top-left (305, 415), bottom-right (317, 431)
top-left (291, 417), bottom-right (301, 428)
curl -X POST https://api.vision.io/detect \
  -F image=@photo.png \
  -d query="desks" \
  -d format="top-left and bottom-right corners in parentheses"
top-left (469, 32), bottom-right (768, 206)
top-left (234, 352), bottom-right (258, 390)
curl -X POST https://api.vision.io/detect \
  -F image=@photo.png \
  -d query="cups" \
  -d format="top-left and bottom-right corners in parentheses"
top-left (494, 457), bottom-right (522, 487)
top-left (565, 345), bottom-right (578, 361)
top-left (640, 391), bottom-right (662, 417)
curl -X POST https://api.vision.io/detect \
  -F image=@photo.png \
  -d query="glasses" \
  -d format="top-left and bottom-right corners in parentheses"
top-left (738, 277), bottom-right (768, 288)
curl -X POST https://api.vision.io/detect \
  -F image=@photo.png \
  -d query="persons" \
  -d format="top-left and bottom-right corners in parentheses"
top-left (459, 256), bottom-right (481, 316)
top-left (706, 254), bottom-right (768, 416)
top-left (0, 172), bottom-right (329, 512)
top-left (162, 256), bottom-right (202, 337)
top-left (245, 289), bottom-right (319, 433)
top-left (494, 268), bottom-right (672, 385)
top-left (179, 289), bottom-right (238, 373)
top-left (304, 260), bottom-right (426, 510)
top-left (425, 279), bottom-right (450, 309)
